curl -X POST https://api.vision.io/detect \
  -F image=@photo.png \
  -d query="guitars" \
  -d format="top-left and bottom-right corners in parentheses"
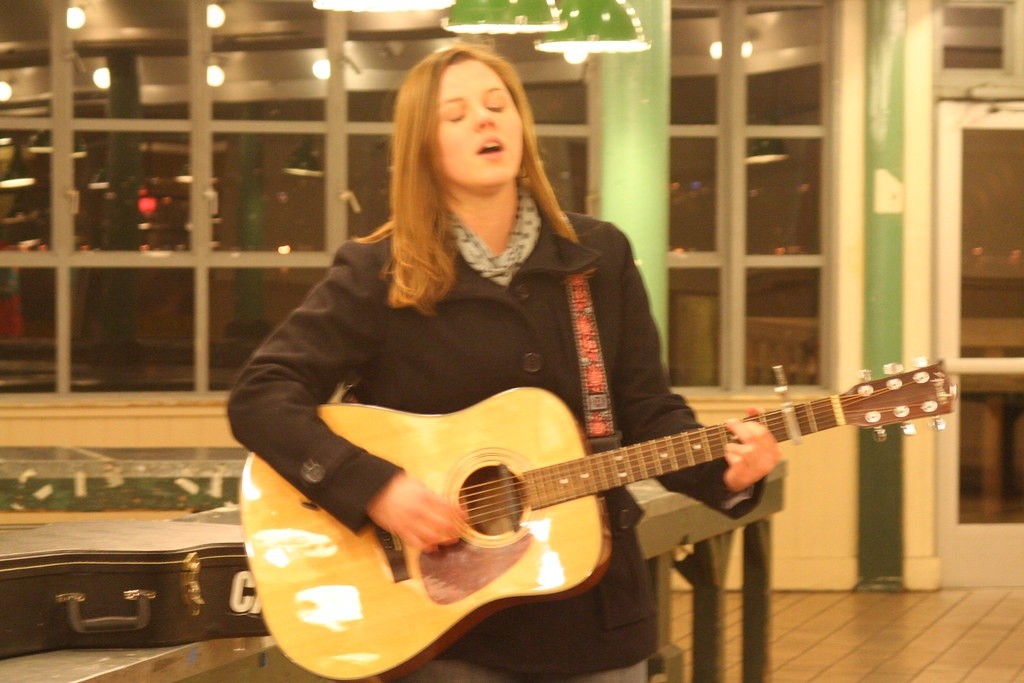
top-left (239, 354), bottom-right (958, 683)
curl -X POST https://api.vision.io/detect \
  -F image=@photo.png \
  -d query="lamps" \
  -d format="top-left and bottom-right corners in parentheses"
top-left (280, 134), bottom-right (324, 179)
top-left (746, 118), bottom-right (790, 164)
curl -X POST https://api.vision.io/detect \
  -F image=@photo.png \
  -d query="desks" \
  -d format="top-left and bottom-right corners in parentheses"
top-left (0, 445), bottom-right (788, 683)
top-left (959, 317), bottom-right (1024, 493)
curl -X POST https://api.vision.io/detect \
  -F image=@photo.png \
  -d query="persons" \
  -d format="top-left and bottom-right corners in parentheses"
top-left (228, 46), bottom-right (780, 683)
